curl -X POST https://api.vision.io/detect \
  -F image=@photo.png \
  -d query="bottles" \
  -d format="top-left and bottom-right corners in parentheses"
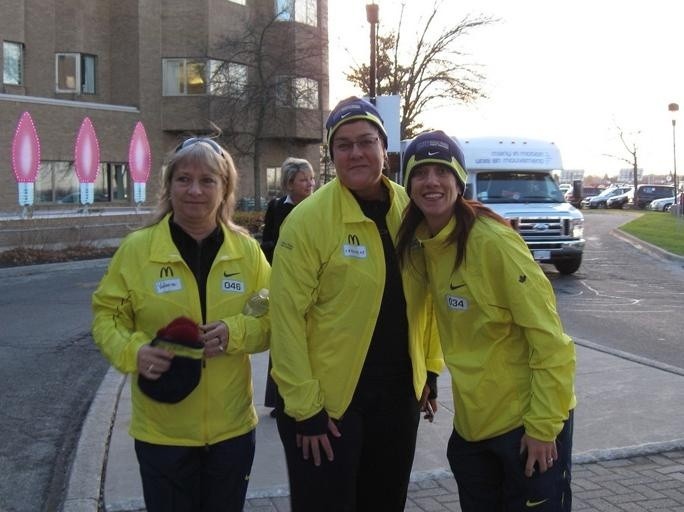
top-left (241, 287), bottom-right (269, 316)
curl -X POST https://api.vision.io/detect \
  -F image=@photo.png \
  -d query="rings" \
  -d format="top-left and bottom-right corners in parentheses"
top-left (147, 364), bottom-right (154, 373)
top-left (214, 336), bottom-right (221, 345)
top-left (546, 457), bottom-right (554, 464)
top-left (219, 345), bottom-right (224, 350)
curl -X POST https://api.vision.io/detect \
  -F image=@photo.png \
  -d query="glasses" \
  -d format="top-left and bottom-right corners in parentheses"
top-left (333, 134), bottom-right (381, 153)
top-left (172, 136), bottom-right (226, 161)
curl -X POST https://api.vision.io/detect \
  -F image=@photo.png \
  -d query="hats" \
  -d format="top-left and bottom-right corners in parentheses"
top-left (397, 130), bottom-right (469, 199)
top-left (324, 96), bottom-right (390, 163)
top-left (138, 314), bottom-right (208, 407)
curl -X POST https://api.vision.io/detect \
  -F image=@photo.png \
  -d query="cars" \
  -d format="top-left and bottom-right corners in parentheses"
top-left (55, 192), bottom-right (108, 202)
top-left (556, 181), bottom-right (684, 212)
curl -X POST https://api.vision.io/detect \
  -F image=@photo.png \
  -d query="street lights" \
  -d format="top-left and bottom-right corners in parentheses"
top-left (668, 102), bottom-right (681, 206)
top-left (367, 1), bottom-right (381, 108)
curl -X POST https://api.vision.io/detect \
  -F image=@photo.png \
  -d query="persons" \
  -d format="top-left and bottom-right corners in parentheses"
top-left (90, 136), bottom-right (272, 511)
top-left (394, 130), bottom-right (577, 511)
top-left (260, 156), bottom-right (316, 419)
top-left (267, 95), bottom-right (444, 511)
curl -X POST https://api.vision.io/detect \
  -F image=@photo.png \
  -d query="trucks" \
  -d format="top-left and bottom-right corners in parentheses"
top-left (452, 136), bottom-right (583, 275)
